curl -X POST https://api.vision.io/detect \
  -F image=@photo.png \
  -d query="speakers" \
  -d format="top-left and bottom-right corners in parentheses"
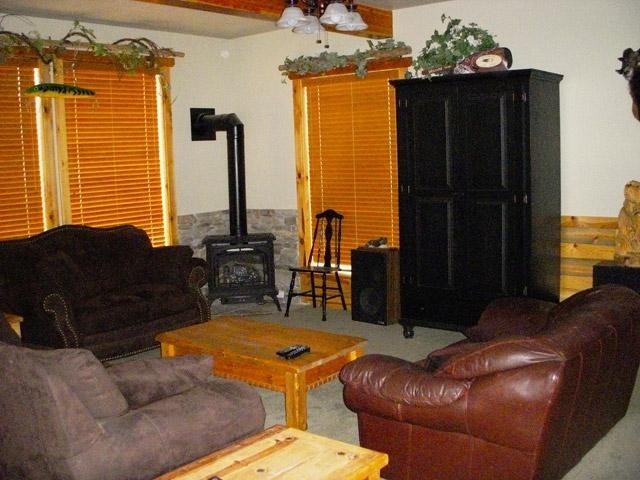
top-left (351, 247), bottom-right (401, 326)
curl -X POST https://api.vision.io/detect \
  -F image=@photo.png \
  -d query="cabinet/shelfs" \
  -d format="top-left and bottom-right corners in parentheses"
top-left (593, 260), bottom-right (639, 293)
top-left (386, 68), bottom-right (564, 341)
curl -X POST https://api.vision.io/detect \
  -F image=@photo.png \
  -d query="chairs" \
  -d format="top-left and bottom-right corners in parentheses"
top-left (1, 340), bottom-right (268, 480)
top-left (283, 209), bottom-right (346, 321)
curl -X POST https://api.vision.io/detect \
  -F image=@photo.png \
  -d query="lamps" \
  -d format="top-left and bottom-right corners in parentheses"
top-left (274, 1), bottom-right (374, 49)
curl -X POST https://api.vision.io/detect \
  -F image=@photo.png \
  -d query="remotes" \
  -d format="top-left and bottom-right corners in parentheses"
top-left (276, 345), bottom-right (301, 357)
top-left (285, 345), bottom-right (310, 360)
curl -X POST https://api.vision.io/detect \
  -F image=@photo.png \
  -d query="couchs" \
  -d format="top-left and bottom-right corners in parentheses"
top-left (1, 223), bottom-right (214, 361)
top-left (337, 281), bottom-right (640, 480)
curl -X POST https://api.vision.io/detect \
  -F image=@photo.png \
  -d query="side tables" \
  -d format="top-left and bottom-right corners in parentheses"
top-left (158, 420), bottom-right (389, 477)
